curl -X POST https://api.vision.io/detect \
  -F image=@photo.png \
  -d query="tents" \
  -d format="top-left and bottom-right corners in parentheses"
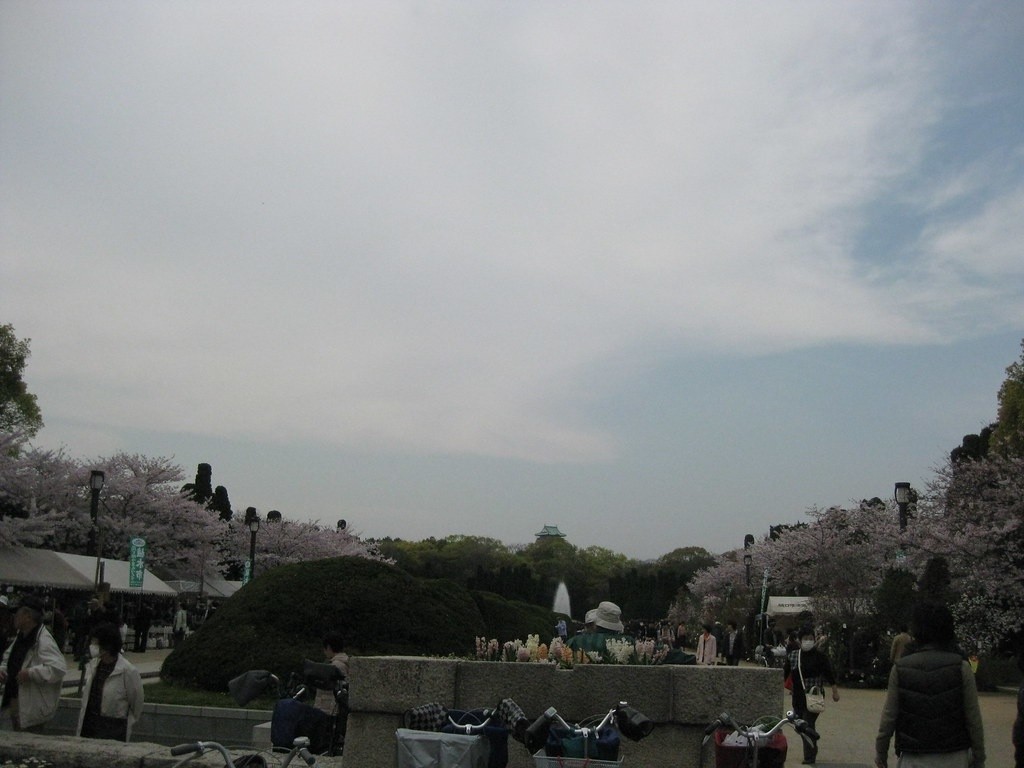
top-left (0, 543), bottom-right (248, 621)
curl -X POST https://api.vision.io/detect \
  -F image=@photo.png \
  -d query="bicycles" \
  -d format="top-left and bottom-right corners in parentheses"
top-left (394, 697), bottom-right (531, 768)
top-left (525, 699), bottom-right (655, 768)
top-left (166, 737), bottom-right (317, 768)
top-left (701, 708), bottom-right (821, 768)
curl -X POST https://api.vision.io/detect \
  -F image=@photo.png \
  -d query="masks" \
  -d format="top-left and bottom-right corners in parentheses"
top-left (89, 644), bottom-right (100, 658)
top-left (800, 640), bottom-right (815, 651)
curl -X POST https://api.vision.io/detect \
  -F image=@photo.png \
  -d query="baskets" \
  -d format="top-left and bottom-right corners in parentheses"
top-left (714, 726), bottom-right (788, 768)
top-left (534, 747), bottom-right (625, 767)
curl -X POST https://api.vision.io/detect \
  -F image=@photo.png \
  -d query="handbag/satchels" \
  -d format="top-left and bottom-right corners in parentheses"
top-left (721, 716), bottom-right (781, 747)
top-left (80, 712), bottom-right (127, 742)
top-left (172, 624), bottom-right (183, 635)
top-left (805, 686), bottom-right (826, 713)
top-left (784, 673), bottom-right (793, 690)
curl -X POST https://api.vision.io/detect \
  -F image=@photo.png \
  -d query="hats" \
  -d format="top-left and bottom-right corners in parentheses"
top-left (585, 601), bottom-right (624, 631)
top-left (8, 596), bottom-right (45, 617)
top-left (0, 595), bottom-right (8, 605)
top-left (87, 598), bottom-right (98, 604)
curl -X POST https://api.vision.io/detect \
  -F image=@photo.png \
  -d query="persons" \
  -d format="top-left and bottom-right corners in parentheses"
top-left (887, 625), bottom-right (916, 663)
top-left (871, 597), bottom-right (988, 766)
top-left (62, 599), bottom-right (188, 656)
top-left (0, 593), bottom-right (69, 733)
top-left (79, 625), bottom-right (141, 744)
top-left (556, 593), bottom-right (847, 705)
top-left (303, 632), bottom-right (350, 752)
